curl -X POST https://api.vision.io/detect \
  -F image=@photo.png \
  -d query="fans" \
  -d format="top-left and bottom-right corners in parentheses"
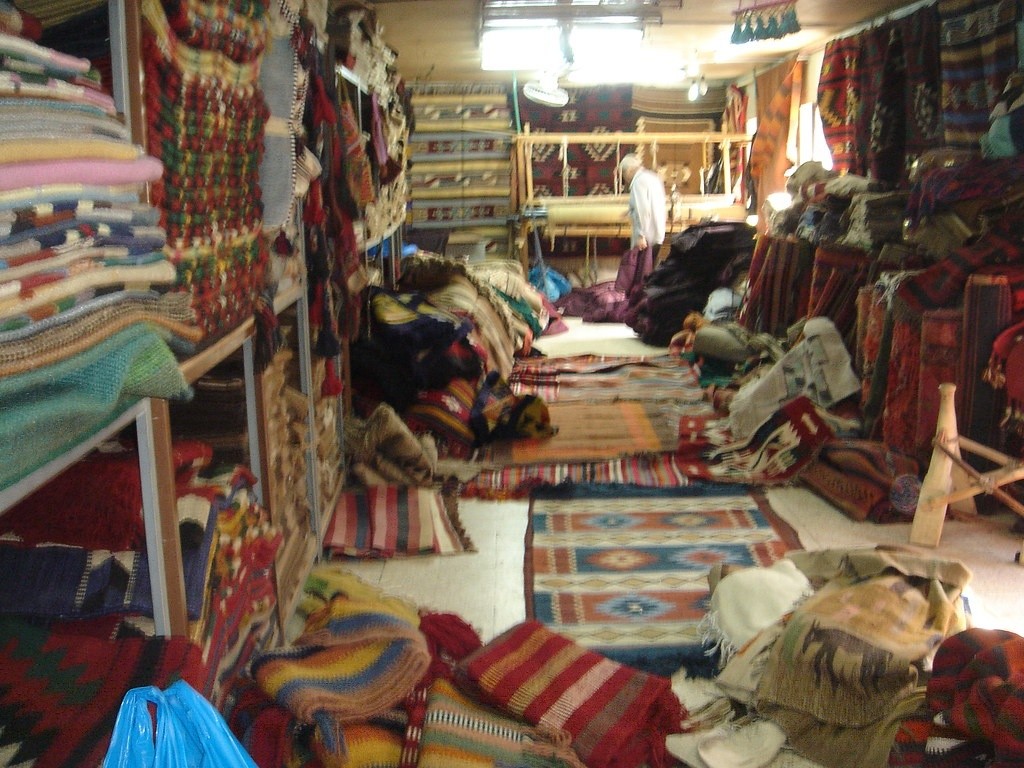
top-left (522, 72), bottom-right (570, 107)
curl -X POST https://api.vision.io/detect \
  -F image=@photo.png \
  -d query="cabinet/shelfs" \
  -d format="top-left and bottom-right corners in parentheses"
top-left (0, 0), bottom-right (407, 768)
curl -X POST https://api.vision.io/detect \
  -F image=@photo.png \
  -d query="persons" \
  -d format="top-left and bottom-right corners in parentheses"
top-left (619, 153), bottom-right (666, 297)
